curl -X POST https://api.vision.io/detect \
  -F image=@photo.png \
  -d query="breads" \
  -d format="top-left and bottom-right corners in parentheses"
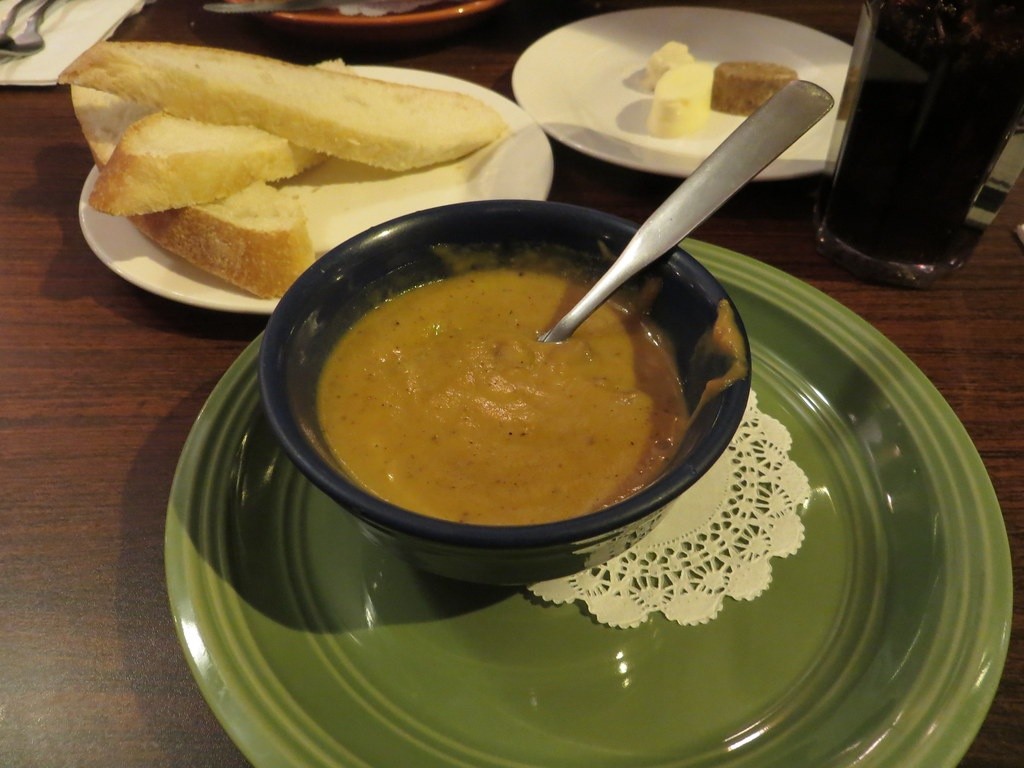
top-left (55, 40), bottom-right (507, 300)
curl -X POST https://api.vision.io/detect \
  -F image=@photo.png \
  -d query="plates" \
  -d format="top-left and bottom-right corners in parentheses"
top-left (164, 236), bottom-right (1014, 768)
top-left (0, 0), bottom-right (156, 87)
top-left (78, 66), bottom-right (554, 315)
top-left (511, 6), bottom-right (854, 182)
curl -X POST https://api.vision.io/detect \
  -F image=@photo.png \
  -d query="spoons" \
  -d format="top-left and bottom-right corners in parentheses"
top-left (0, 0), bottom-right (56, 57)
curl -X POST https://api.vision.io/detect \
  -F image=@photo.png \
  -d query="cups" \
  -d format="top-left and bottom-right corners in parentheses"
top-left (815, 0), bottom-right (1024, 290)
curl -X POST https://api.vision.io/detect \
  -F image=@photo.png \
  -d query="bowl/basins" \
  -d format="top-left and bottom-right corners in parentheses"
top-left (259, 198), bottom-right (752, 589)
top-left (223, 0), bottom-right (503, 48)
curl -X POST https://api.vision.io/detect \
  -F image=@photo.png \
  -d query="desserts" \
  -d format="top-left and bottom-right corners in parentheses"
top-left (644, 39), bottom-right (799, 139)
